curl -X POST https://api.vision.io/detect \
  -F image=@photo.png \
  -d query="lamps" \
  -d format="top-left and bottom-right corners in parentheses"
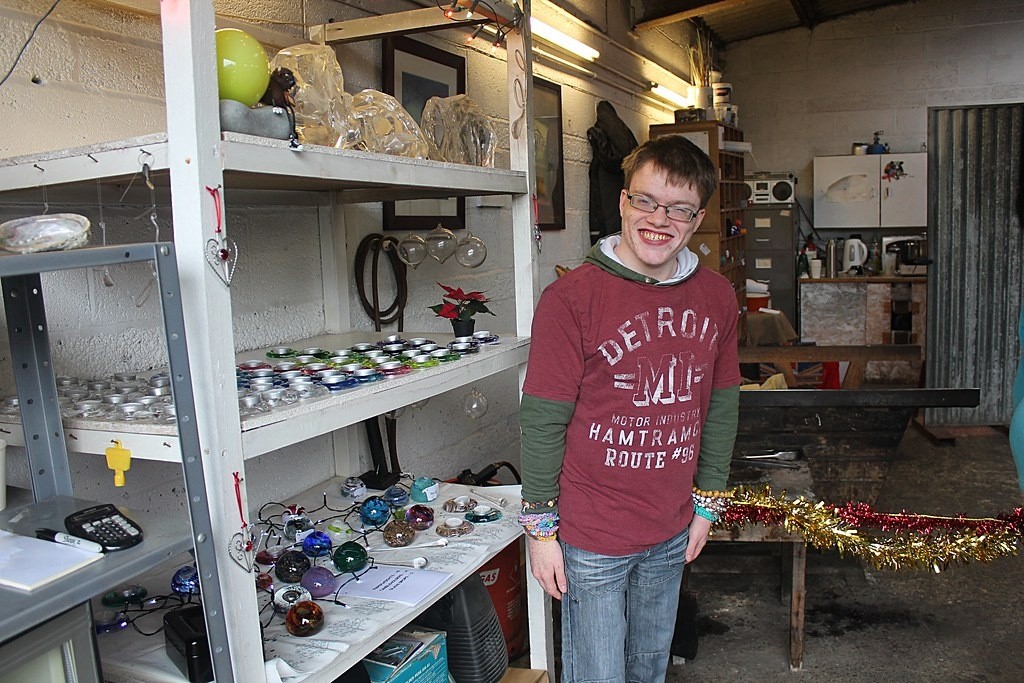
top-left (530, 16), bottom-right (602, 63)
top-left (647, 81), bottom-right (693, 107)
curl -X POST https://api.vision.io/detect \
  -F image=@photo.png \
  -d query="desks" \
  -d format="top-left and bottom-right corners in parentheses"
top-left (798, 275), bottom-right (928, 386)
top-left (705, 445), bottom-right (814, 669)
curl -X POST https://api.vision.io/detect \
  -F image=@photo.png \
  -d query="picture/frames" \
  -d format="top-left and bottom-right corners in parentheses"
top-left (532, 75), bottom-right (566, 231)
top-left (382, 34), bottom-right (466, 231)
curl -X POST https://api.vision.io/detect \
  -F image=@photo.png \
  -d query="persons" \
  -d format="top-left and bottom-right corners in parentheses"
top-left (519, 135), bottom-right (740, 683)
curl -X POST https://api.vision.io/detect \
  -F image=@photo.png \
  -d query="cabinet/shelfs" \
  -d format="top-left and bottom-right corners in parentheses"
top-left (813, 151), bottom-right (928, 230)
top-left (0, 0), bottom-right (555, 683)
top-left (649, 119), bottom-right (749, 346)
top-left (748, 204), bottom-right (801, 347)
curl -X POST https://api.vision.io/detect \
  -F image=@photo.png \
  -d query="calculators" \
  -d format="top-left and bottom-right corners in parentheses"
top-left (65, 504), bottom-right (143, 552)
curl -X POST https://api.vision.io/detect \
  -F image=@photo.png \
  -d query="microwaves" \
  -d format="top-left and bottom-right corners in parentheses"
top-left (881, 235), bottom-right (927, 275)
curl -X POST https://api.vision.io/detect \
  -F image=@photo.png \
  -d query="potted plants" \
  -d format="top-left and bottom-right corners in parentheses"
top-left (685, 27), bottom-right (714, 109)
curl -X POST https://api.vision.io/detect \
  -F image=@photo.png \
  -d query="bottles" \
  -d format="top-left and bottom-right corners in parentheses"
top-left (827, 239), bottom-right (837, 278)
top-left (870, 236), bottom-right (879, 258)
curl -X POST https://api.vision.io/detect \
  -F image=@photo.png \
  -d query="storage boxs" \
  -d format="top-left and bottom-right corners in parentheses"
top-left (332, 625), bottom-right (447, 683)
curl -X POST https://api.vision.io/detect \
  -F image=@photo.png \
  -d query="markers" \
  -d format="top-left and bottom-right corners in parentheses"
top-left (35, 528), bottom-right (102, 552)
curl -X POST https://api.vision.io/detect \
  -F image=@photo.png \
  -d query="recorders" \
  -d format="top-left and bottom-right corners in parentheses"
top-left (743, 170), bottom-right (798, 204)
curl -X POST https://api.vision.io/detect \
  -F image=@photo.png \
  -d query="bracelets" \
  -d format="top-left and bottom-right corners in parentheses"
top-left (519, 499), bottom-right (559, 540)
top-left (692, 487), bottom-right (729, 522)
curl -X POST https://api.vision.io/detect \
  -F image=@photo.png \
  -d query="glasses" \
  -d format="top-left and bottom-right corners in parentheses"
top-left (626, 193), bottom-right (697, 222)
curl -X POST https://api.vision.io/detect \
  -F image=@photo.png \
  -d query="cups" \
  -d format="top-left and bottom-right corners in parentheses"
top-left (883, 254), bottom-right (896, 275)
top-left (811, 260), bottom-right (822, 278)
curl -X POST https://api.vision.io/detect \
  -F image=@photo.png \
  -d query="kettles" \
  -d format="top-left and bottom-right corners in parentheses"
top-left (843, 239), bottom-right (868, 272)
